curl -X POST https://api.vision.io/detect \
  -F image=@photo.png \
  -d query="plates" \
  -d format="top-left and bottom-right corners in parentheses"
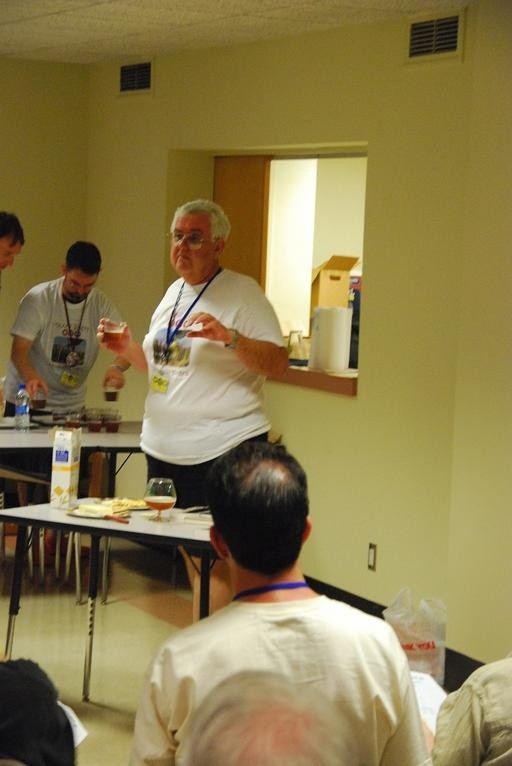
top-left (66, 495), bottom-right (149, 520)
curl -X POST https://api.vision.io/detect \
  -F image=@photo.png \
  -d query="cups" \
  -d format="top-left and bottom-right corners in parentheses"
top-left (33, 387), bottom-right (47, 410)
top-left (288, 328), bottom-right (308, 359)
top-left (104, 382), bottom-right (119, 401)
top-left (53, 406), bottom-right (124, 432)
top-left (310, 308), bottom-right (354, 374)
top-left (101, 318), bottom-right (127, 347)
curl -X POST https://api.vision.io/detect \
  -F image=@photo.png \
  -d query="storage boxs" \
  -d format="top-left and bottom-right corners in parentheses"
top-left (310, 256), bottom-right (359, 320)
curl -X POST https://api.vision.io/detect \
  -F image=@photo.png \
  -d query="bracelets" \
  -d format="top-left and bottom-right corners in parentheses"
top-left (109, 362), bottom-right (126, 371)
top-left (224, 328), bottom-right (239, 352)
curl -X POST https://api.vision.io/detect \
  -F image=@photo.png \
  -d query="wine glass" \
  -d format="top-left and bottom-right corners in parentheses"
top-left (1, 376), bottom-right (8, 424)
top-left (143, 476), bottom-right (178, 523)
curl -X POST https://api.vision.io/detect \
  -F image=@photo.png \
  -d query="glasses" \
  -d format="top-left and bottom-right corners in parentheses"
top-left (165, 229), bottom-right (216, 250)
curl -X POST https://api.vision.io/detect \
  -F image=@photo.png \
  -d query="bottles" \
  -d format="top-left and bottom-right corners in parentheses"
top-left (14, 383), bottom-right (30, 433)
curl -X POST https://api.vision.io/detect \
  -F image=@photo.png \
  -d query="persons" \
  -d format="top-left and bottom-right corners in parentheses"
top-left (176, 671), bottom-right (364, 766)
top-left (431, 657), bottom-right (512, 766)
top-left (96, 199), bottom-right (289, 624)
top-left (134, 440), bottom-right (434, 766)
top-left (3, 241), bottom-right (131, 567)
top-left (0, 213), bottom-right (24, 272)
